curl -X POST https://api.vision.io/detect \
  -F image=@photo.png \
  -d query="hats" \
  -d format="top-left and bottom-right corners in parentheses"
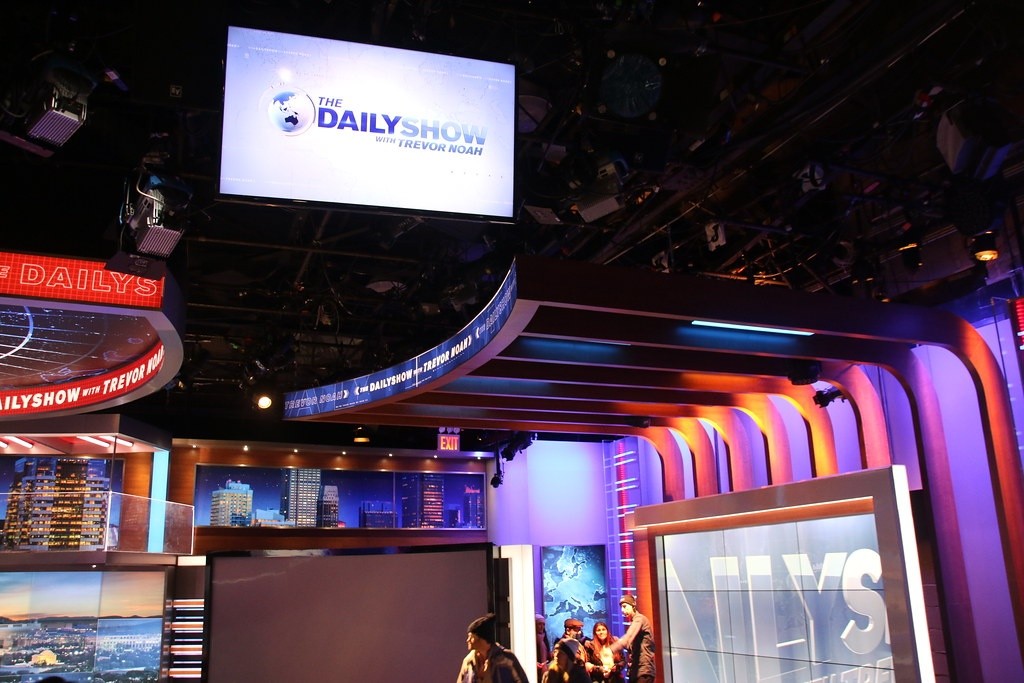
top-left (565, 618), bottom-right (583, 630)
top-left (468, 613), bottom-right (496, 647)
top-left (554, 638), bottom-right (579, 663)
top-left (535, 613), bottom-right (544, 622)
top-left (619, 595), bottom-right (634, 606)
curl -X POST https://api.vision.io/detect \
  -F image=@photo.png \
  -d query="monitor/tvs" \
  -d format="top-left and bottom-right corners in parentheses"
top-left (213, 23), bottom-right (519, 225)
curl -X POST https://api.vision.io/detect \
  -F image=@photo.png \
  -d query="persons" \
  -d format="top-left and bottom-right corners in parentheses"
top-left (553, 619), bottom-right (627, 683)
top-left (604, 594), bottom-right (656, 683)
top-left (541, 638), bottom-right (591, 683)
top-left (535, 613), bottom-right (552, 683)
top-left (457, 613), bottom-right (529, 683)
